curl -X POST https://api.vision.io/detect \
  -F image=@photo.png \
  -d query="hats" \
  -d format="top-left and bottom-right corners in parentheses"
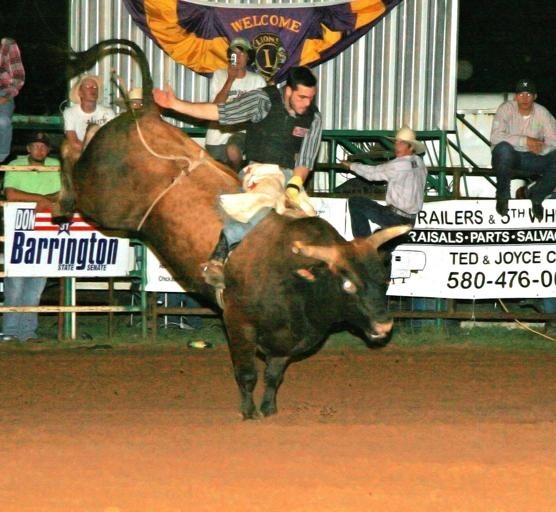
top-left (515, 79), bottom-right (536, 94)
top-left (116, 88), bottom-right (145, 108)
top-left (68, 75), bottom-right (105, 104)
top-left (384, 126), bottom-right (425, 155)
top-left (229, 37), bottom-right (252, 51)
top-left (24, 131), bottom-right (49, 147)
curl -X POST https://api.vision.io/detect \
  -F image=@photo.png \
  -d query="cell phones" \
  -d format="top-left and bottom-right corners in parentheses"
top-left (231, 54), bottom-right (237, 66)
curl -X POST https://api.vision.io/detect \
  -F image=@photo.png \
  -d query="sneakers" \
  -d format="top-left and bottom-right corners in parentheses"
top-left (198, 259), bottom-right (226, 290)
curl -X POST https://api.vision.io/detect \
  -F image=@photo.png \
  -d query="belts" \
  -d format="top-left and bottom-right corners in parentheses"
top-left (390, 206), bottom-right (416, 220)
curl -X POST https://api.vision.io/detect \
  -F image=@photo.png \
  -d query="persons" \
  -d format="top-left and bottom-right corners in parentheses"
top-left (200, 36), bottom-right (267, 176)
top-left (146, 292), bottom-right (205, 332)
top-left (113, 86), bottom-right (146, 115)
top-left (150, 65), bottom-right (323, 285)
top-left (490, 78), bottom-right (556, 217)
top-left (335, 123), bottom-right (430, 296)
top-left (0, 130), bottom-right (69, 345)
top-left (53, 74), bottom-right (118, 217)
top-left (0, 34), bottom-right (28, 179)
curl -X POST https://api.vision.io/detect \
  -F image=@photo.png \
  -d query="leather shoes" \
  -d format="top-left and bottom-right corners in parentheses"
top-left (27, 337), bottom-right (42, 343)
top-left (496, 198), bottom-right (507, 215)
top-left (532, 204), bottom-right (543, 220)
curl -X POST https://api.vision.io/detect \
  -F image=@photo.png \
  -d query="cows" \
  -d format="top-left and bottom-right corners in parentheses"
top-left (22, 36), bottom-right (415, 422)
